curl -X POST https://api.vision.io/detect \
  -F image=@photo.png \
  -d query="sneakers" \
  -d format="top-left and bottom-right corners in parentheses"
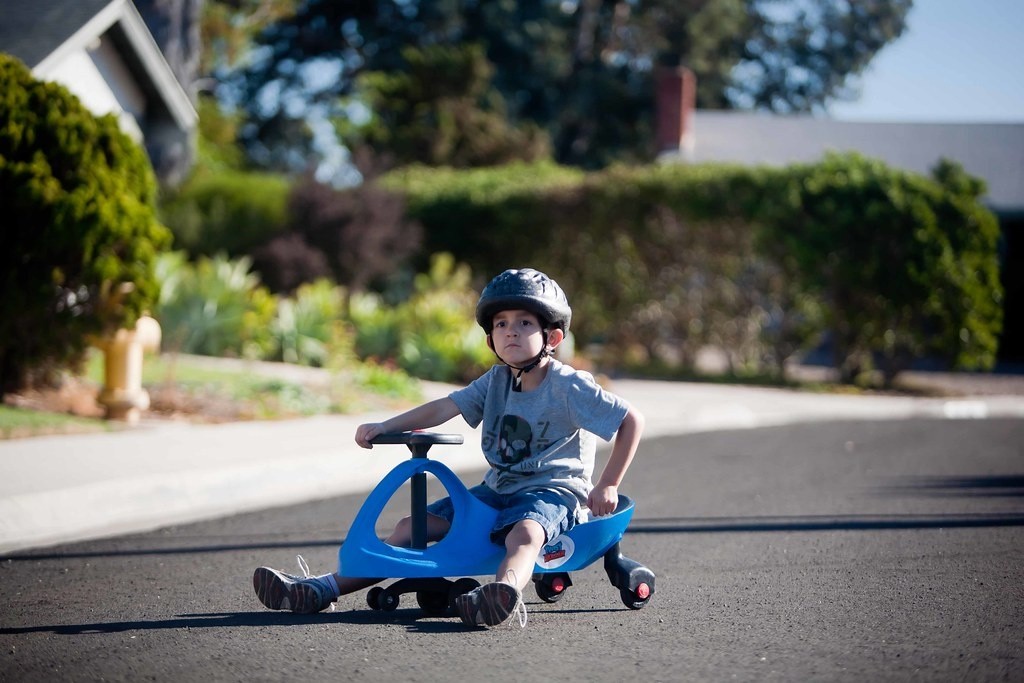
top-left (455, 581), bottom-right (523, 627)
top-left (252, 567), bottom-right (334, 616)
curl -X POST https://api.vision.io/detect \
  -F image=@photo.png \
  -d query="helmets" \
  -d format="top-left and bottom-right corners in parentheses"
top-left (475, 268), bottom-right (573, 339)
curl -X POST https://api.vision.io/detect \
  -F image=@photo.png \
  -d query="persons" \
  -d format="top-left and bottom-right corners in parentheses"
top-left (253, 268), bottom-right (644, 626)
